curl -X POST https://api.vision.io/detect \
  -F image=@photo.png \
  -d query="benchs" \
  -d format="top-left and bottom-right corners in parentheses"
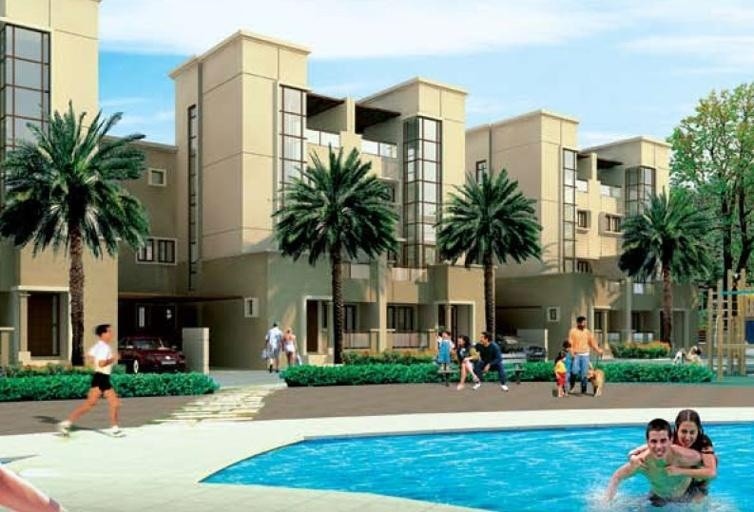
top-left (436, 352), bottom-right (527, 386)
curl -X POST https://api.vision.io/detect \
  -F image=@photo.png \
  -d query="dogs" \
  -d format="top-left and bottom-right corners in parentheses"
top-left (585, 368), bottom-right (605, 396)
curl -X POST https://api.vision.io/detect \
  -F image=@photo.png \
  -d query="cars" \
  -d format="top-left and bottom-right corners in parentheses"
top-left (495, 331), bottom-right (547, 362)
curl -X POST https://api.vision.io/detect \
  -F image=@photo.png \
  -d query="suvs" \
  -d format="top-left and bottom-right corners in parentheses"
top-left (116, 334), bottom-right (187, 374)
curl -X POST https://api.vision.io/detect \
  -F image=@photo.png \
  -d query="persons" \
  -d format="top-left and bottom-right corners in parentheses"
top-left (1, 468), bottom-right (66, 511)
top-left (55, 324), bottom-right (126, 437)
top-left (265, 320), bottom-right (283, 372)
top-left (626, 411), bottom-right (718, 495)
top-left (607, 419), bottom-right (707, 506)
top-left (554, 316), bottom-right (605, 400)
top-left (282, 327), bottom-right (298, 366)
top-left (683, 344), bottom-right (704, 369)
top-left (433, 327), bottom-right (515, 390)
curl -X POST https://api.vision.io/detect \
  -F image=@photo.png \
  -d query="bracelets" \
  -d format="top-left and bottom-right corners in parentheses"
top-left (628, 454), bottom-right (634, 458)
top-left (106, 360), bottom-right (109, 365)
